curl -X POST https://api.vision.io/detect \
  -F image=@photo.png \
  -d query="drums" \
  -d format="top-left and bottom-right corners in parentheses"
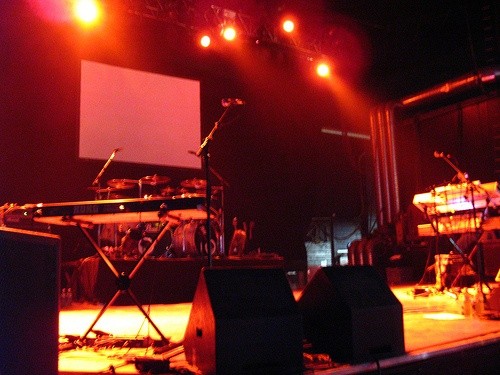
top-left (95, 188), bottom-right (135, 200)
top-left (138, 174), bottom-right (177, 197)
top-left (171, 218), bottom-right (223, 258)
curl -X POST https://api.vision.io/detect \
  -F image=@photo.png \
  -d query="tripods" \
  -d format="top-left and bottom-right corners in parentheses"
top-left (416, 158), bottom-right (493, 296)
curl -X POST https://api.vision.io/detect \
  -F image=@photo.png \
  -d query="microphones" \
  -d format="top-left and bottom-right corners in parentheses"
top-left (208, 100), bottom-right (241, 141)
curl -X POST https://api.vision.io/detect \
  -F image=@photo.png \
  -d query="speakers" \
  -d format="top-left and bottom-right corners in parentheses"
top-left (297, 265), bottom-right (406, 366)
top-left (0, 227), bottom-right (60, 375)
top-left (184, 265), bottom-right (304, 375)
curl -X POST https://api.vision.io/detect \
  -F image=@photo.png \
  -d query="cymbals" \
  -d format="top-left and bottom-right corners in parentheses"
top-left (106, 178), bottom-right (139, 189)
top-left (178, 179), bottom-right (208, 195)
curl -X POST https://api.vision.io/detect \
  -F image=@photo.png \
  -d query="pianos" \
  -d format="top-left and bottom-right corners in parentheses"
top-left (32, 196), bottom-right (223, 348)
top-left (412, 180), bottom-right (500, 292)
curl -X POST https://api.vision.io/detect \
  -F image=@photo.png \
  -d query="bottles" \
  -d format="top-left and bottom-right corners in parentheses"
top-left (475, 288), bottom-right (484, 314)
top-left (463, 287), bottom-right (471, 317)
